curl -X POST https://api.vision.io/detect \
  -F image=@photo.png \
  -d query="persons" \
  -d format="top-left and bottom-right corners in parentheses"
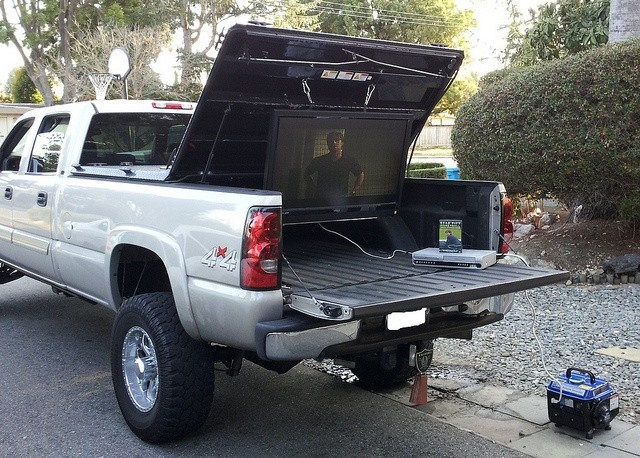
top-left (303, 132), bottom-right (365, 198)
top-left (445, 230), bottom-right (462, 249)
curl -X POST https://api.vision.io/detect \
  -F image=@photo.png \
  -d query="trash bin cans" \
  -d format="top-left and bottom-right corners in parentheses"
top-left (447, 168), bottom-right (460, 180)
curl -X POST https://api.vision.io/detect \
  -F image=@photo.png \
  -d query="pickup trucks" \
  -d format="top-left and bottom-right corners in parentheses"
top-left (0, 20), bottom-right (571, 448)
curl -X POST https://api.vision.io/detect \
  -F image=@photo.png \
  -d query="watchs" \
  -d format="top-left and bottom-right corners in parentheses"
top-left (351, 190), bottom-right (357, 195)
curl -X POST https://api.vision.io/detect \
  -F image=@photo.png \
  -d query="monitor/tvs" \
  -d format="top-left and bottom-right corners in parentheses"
top-left (266, 111), bottom-right (414, 228)
